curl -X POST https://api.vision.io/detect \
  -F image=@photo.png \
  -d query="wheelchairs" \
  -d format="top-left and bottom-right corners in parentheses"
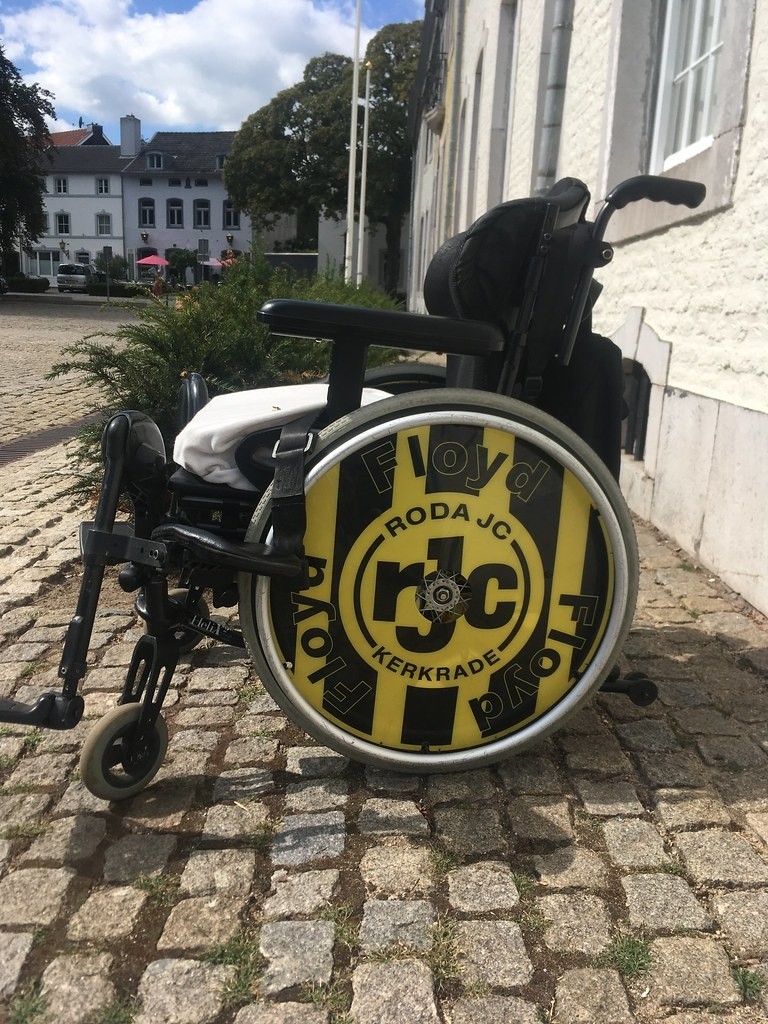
top-left (0, 172), bottom-right (707, 802)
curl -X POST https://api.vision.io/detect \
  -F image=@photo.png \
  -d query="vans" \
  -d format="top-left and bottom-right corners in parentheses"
top-left (56, 263), bottom-right (99, 293)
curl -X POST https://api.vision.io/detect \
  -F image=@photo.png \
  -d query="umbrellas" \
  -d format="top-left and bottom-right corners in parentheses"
top-left (135, 255), bottom-right (169, 282)
top-left (200, 257), bottom-right (222, 269)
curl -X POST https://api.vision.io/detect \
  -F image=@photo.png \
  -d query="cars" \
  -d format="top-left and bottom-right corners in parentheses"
top-left (0, 278), bottom-right (9, 295)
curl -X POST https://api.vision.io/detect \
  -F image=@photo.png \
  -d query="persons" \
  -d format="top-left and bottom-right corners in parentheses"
top-left (153, 270), bottom-right (164, 299)
